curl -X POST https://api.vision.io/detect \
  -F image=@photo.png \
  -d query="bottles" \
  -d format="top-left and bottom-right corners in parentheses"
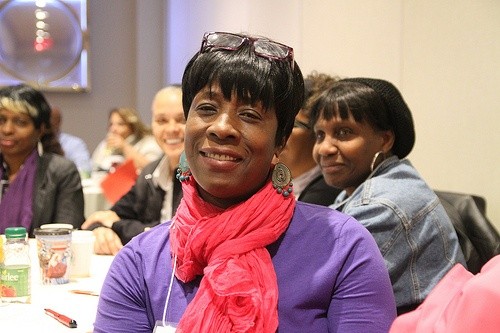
top-left (0, 227), bottom-right (32, 306)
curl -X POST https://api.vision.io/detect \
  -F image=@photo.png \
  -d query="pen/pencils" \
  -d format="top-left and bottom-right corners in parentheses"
top-left (44, 307), bottom-right (77, 327)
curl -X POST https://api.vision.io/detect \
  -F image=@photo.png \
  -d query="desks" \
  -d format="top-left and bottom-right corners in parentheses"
top-left (0, 230), bottom-right (113, 333)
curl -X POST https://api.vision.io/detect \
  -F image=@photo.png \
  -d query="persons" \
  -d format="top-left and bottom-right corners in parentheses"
top-left (389, 255), bottom-right (500, 333)
top-left (307, 78), bottom-right (466, 316)
top-left (80, 83), bottom-right (187, 256)
top-left (0, 83), bottom-right (85, 238)
top-left (271, 70), bottom-right (343, 206)
top-left (90, 106), bottom-right (154, 180)
top-left (37, 105), bottom-right (88, 179)
top-left (93, 30), bottom-right (397, 333)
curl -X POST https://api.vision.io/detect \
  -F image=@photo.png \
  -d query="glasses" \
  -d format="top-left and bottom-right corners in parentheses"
top-left (201, 32), bottom-right (294, 75)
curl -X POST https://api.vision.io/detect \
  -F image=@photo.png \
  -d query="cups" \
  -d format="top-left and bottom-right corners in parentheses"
top-left (33, 223), bottom-right (96, 285)
